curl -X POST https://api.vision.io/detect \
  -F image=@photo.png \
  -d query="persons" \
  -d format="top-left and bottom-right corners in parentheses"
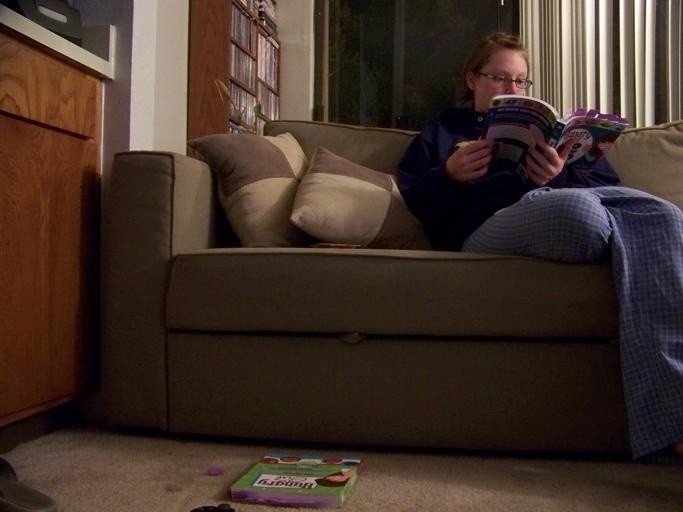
top-left (397, 26), bottom-right (683, 459)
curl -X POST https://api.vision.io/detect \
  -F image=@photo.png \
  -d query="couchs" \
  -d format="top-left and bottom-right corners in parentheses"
top-left (93, 118), bottom-right (680, 452)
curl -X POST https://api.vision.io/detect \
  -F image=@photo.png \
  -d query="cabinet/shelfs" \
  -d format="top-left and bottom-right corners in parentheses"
top-left (0, 31), bottom-right (106, 435)
top-left (188, 0), bottom-right (281, 158)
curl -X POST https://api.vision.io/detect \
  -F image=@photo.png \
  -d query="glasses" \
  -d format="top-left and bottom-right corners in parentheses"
top-left (471, 69), bottom-right (534, 89)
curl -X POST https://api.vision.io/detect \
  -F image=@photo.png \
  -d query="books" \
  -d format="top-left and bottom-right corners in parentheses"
top-left (229, 1), bottom-right (279, 137)
top-left (480, 95), bottom-right (628, 180)
top-left (228, 450), bottom-right (367, 506)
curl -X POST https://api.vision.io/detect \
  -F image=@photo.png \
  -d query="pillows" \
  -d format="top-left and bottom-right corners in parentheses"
top-left (183, 129), bottom-right (426, 253)
